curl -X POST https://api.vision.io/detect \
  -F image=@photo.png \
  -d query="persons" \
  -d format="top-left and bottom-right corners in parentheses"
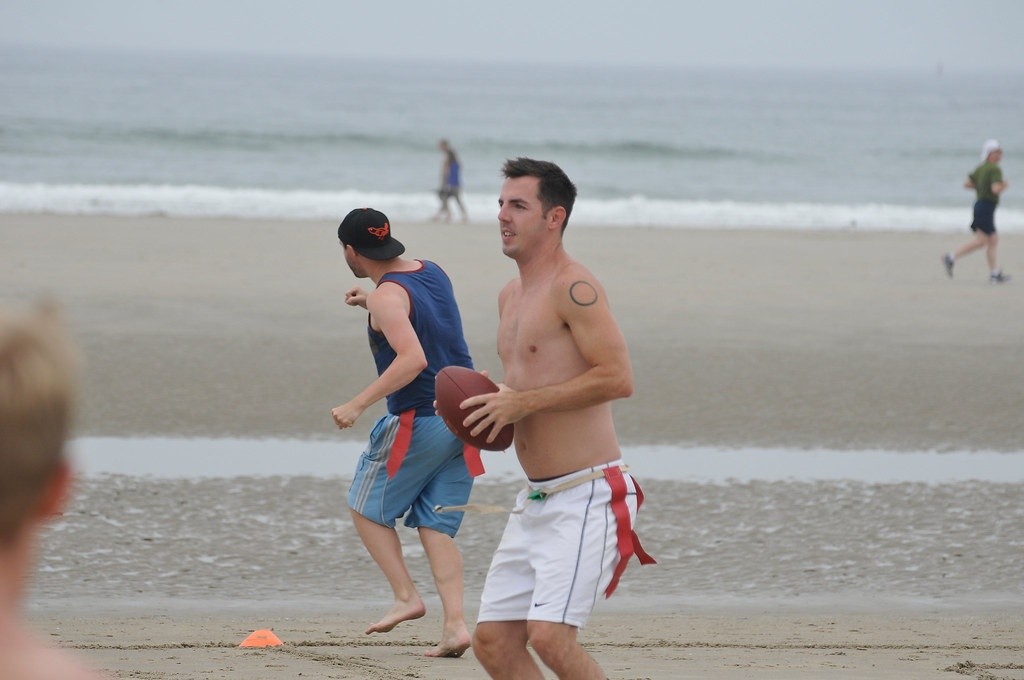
top-left (331, 208), bottom-right (482, 656)
top-left (432, 138), bottom-right (470, 223)
top-left (0, 308), bottom-right (110, 680)
top-left (944, 140), bottom-right (1010, 284)
top-left (433, 158), bottom-right (656, 680)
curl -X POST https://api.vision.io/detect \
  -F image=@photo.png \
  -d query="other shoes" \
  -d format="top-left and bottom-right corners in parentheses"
top-left (991, 273), bottom-right (1011, 283)
top-left (941, 254), bottom-right (954, 277)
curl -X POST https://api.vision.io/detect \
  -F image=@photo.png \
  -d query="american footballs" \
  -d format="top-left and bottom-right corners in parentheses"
top-left (435, 366), bottom-right (514, 452)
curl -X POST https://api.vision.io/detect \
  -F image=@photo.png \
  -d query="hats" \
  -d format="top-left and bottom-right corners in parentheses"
top-left (338, 208), bottom-right (405, 260)
top-left (982, 140), bottom-right (1000, 161)
